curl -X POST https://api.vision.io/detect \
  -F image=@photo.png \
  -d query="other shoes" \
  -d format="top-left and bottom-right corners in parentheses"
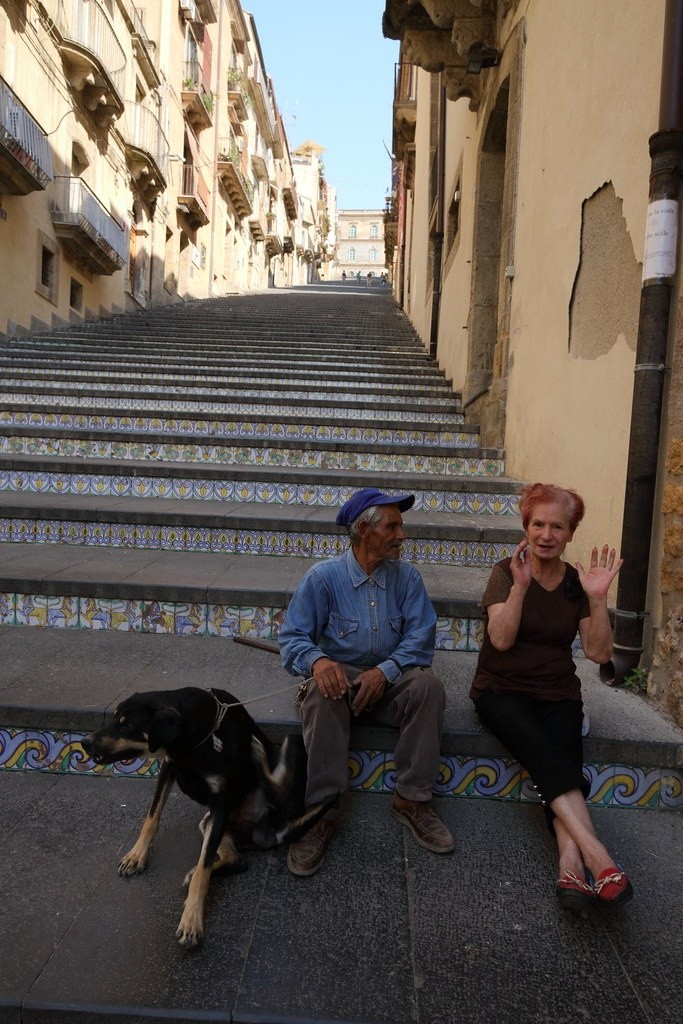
top-left (597, 863), bottom-right (635, 911)
top-left (556, 871), bottom-right (595, 915)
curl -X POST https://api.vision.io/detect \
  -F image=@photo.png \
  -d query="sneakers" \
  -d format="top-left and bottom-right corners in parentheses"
top-left (390, 791), bottom-right (456, 854)
top-left (287, 802), bottom-right (342, 877)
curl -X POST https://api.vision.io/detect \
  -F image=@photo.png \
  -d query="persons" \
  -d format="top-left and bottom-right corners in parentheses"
top-left (367, 272), bottom-right (372, 286)
top-left (277, 489), bottom-right (455, 877)
top-left (468, 482), bottom-right (632, 911)
top-left (380, 272), bottom-right (388, 285)
top-left (342, 270), bottom-right (346, 284)
top-left (357, 271), bottom-right (361, 285)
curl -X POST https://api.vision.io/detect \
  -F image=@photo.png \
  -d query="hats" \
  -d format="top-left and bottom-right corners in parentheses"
top-left (334, 488), bottom-right (415, 530)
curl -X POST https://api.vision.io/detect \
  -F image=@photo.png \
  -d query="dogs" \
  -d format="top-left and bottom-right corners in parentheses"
top-left (78, 685), bottom-right (307, 947)
